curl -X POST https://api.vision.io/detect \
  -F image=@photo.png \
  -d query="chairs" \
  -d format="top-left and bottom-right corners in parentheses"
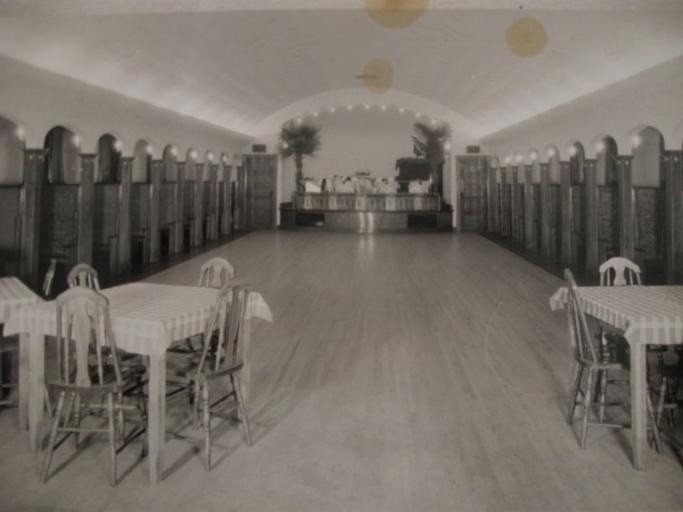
top-left (558, 255), bottom-right (681, 472)
